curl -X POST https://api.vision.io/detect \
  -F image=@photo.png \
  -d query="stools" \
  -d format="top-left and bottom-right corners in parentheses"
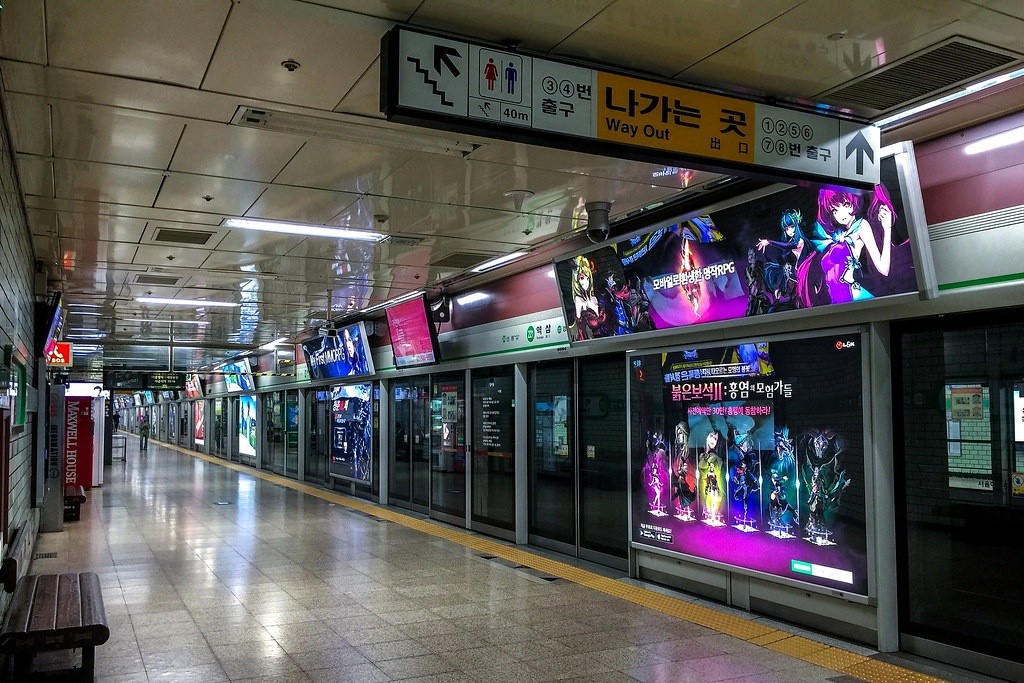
top-left (0, 572), bottom-right (111, 683)
top-left (63, 484), bottom-right (87, 521)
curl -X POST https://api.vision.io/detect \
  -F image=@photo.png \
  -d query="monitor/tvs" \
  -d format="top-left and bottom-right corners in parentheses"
top-left (102, 366), bottom-right (186, 392)
top-left (384, 291), bottom-right (442, 370)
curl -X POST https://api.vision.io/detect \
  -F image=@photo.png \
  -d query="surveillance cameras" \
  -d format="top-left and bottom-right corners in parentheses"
top-left (319, 327), bottom-right (337, 338)
top-left (585, 201), bottom-right (613, 244)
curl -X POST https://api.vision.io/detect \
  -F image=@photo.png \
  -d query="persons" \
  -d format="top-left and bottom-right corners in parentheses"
top-left (215, 415), bottom-right (225, 450)
top-left (93, 386), bottom-right (102, 397)
top-left (139, 416), bottom-right (151, 451)
top-left (113, 411), bottom-right (122, 434)
top-left (139, 305), bottom-right (153, 338)
